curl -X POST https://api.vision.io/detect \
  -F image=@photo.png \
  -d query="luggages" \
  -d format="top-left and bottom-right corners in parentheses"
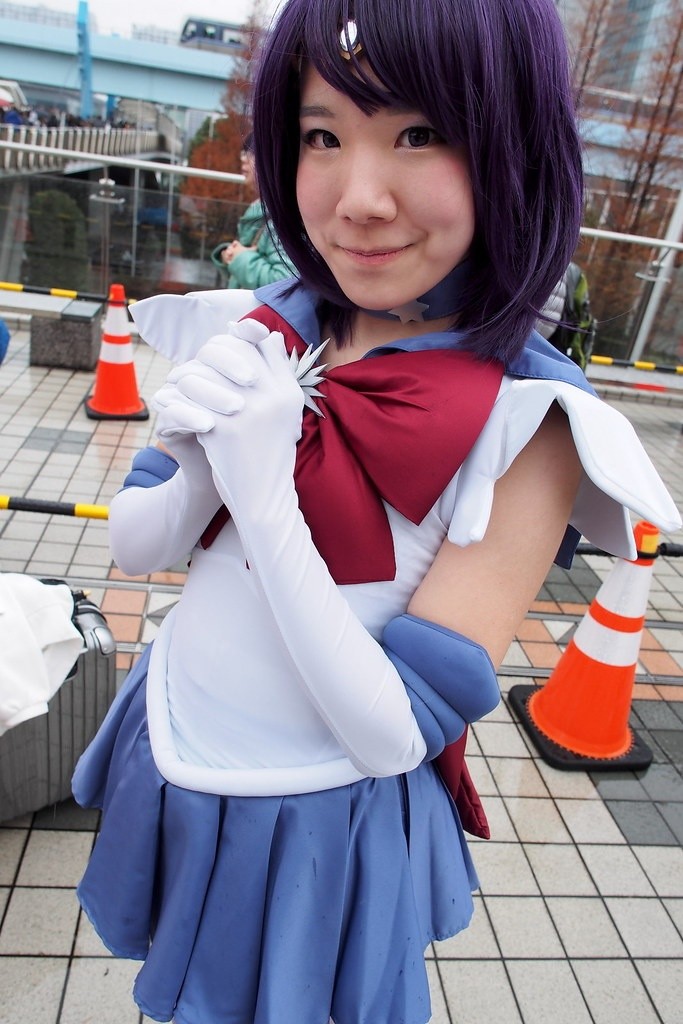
top-left (0, 575), bottom-right (118, 824)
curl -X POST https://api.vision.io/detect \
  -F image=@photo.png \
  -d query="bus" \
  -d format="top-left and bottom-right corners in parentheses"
top-left (567, 84), bottom-right (683, 162)
top-left (182, 15), bottom-right (272, 61)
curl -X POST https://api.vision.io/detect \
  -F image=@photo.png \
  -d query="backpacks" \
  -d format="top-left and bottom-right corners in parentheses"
top-left (550, 261), bottom-right (597, 372)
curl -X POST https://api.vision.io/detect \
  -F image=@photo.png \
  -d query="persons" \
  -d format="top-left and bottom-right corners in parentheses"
top-left (533, 260), bottom-right (589, 354)
top-left (0, 103), bottom-right (136, 128)
top-left (210, 133), bottom-right (301, 288)
top-left (71, 2), bottom-right (682, 1023)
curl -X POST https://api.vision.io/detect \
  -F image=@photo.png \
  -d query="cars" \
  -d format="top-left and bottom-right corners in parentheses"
top-left (134, 202), bottom-right (235, 288)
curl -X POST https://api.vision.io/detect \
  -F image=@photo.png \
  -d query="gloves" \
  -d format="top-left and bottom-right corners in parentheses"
top-left (105, 320), bottom-right (502, 778)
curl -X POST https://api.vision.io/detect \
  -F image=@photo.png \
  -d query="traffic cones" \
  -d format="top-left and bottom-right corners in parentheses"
top-left (84, 283), bottom-right (149, 421)
top-left (507, 520), bottom-right (659, 772)
